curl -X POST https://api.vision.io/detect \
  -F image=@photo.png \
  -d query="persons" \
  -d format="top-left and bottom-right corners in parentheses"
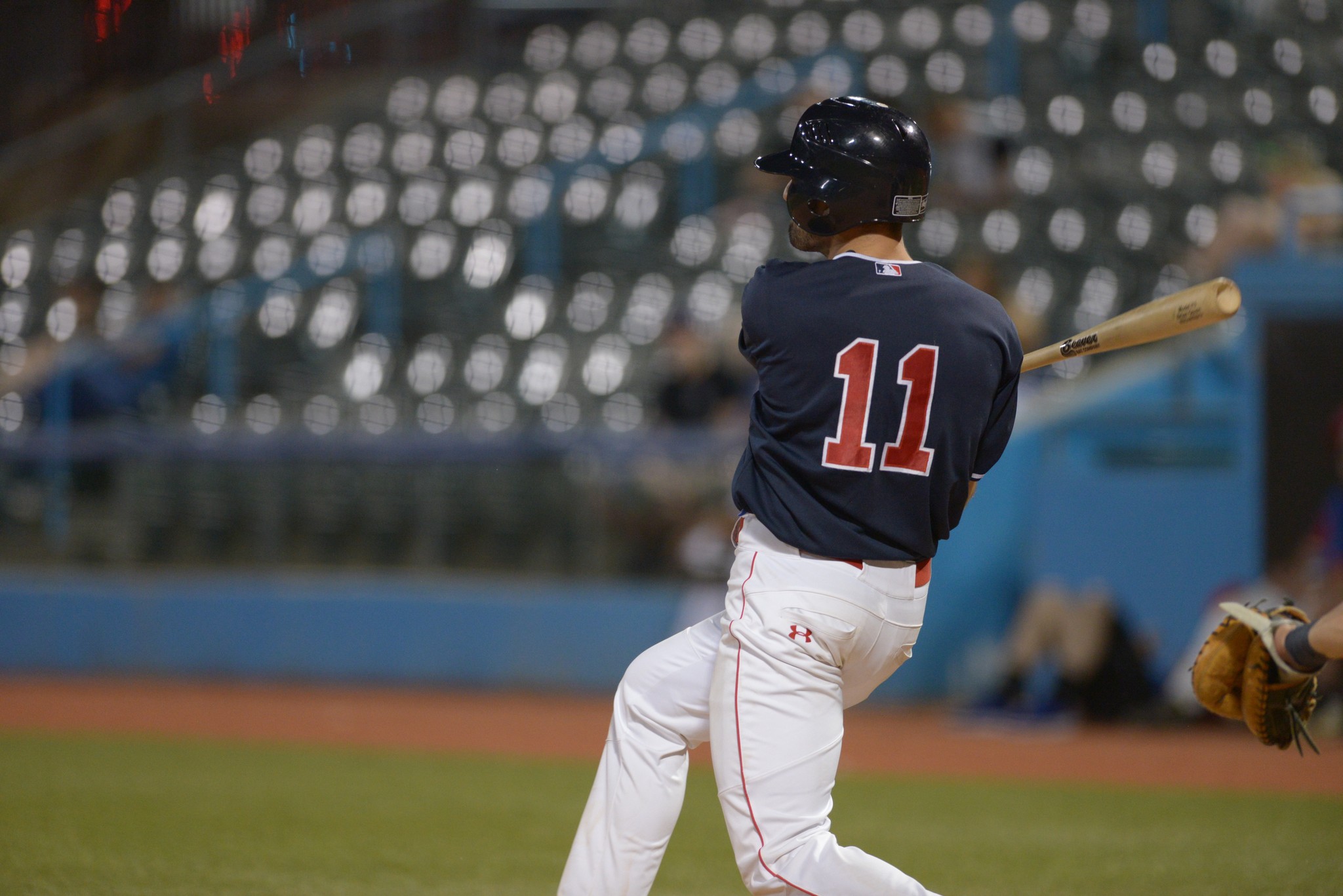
top-left (549, 99), bottom-right (1022, 896)
top-left (1193, 599), bottom-right (1343, 756)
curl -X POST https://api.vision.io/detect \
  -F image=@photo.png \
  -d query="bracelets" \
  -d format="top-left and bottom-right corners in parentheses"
top-left (1284, 621), bottom-right (1330, 670)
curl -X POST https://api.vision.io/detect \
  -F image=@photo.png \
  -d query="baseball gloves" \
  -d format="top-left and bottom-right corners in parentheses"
top-left (1190, 603), bottom-right (1319, 750)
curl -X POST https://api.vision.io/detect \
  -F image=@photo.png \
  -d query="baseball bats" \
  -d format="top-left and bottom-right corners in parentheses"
top-left (1022, 275), bottom-right (1242, 374)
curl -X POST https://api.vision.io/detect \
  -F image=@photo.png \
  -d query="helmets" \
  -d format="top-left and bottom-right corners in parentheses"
top-left (751, 93), bottom-right (933, 235)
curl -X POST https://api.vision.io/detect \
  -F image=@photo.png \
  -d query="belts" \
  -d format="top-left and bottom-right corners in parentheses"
top-left (851, 553), bottom-right (933, 590)
